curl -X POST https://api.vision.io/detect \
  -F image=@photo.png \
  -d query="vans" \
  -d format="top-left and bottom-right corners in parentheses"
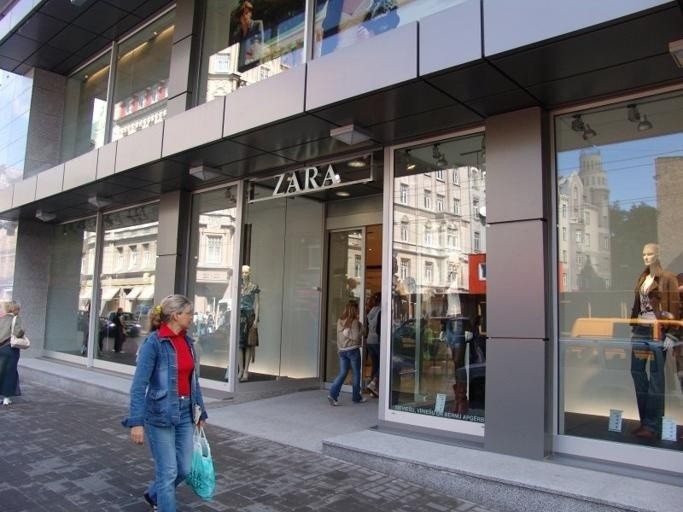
top-left (568, 316), bottom-right (683, 363)
top-left (374, 317), bottom-right (470, 373)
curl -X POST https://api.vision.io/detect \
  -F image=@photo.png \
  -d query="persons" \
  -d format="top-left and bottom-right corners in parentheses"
top-left (236, 265), bottom-right (262, 381)
top-left (0, 301), bottom-right (24, 405)
top-left (321, 0), bottom-right (400, 56)
top-left (630, 243), bottom-right (683, 438)
top-left (367, 292), bottom-right (380, 397)
top-left (439, 253), bottom-right (475, 418)
top-left (120, 294), bottom-right (208, 512)
top-left (112, 307), bottom-right (127, 353)
top-left (231, 0), bottom-right (264, 67)
top-left (328, 300), bottom-right (368, 406)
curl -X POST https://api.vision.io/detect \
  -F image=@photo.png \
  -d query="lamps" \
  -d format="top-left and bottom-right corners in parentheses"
top-left (36, 211), bottom-right (56, 223)
top-left (329, 123), bottom-right (372, 146)
top-left (430, 144), bottom-right (450, 168)
top-left (88, 197), bottom-right (112, 209)
top-left (189, 166), bottom-right (223, 183)
top-left (626, 106), bottom-right (652, 132)
top-left (572, 113), bottom-right (596, 141)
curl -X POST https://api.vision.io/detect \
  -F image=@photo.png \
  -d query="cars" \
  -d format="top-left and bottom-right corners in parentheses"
top-left (77, 309), bottom-right (142, 337)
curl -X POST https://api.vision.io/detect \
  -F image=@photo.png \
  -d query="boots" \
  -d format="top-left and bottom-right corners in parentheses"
top-left (451, 383), bottom-right (469, 413)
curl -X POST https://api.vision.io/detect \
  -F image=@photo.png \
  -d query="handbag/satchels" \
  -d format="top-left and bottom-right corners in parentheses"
top-left (10, 335), bottom-right (30, 350)
top-left (185, 426), bottom-right (216, 501)
top-left (343, 328), bottom-right (353, 339)
top-left (194, 405), bottom-right (201, 424)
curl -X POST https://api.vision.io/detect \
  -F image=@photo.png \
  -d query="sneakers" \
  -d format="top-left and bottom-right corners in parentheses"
top-left (366, 381), bottom-right (379, 397)
top-left (143, 491), bottom-right (157, 512)
top-left (328, 395), bottom-right (338, 406)
top-left (0, 398), bottom-right (12, 406)
